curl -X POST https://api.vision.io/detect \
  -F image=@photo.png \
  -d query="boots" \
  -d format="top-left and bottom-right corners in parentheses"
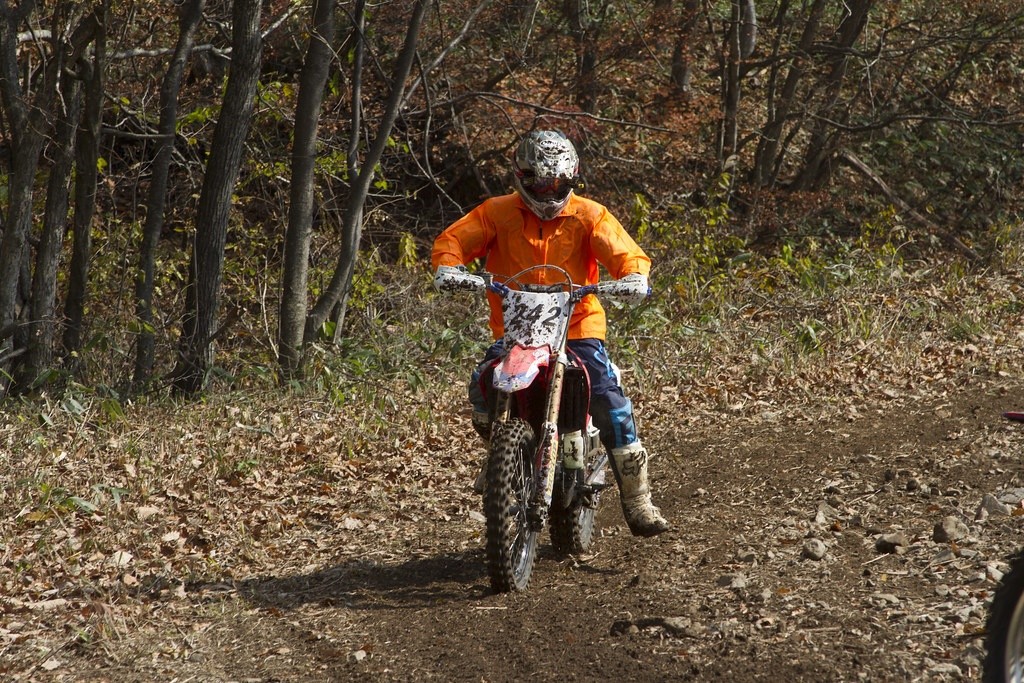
top-left (605, 448), bottom-right (671, 538)
top-left (474, 458), bottom-right (488, 493)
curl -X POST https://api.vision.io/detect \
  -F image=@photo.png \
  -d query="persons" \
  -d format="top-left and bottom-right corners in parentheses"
top-left (431, 131), bottom-right (669, 537)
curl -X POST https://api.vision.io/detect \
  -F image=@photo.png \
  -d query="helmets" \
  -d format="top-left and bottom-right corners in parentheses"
top-left (511, 131), bottom-right (579, 221)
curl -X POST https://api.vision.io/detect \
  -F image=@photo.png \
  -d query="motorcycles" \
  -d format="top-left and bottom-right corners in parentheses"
top-left (443, 267), bottom-right (653, 594)
